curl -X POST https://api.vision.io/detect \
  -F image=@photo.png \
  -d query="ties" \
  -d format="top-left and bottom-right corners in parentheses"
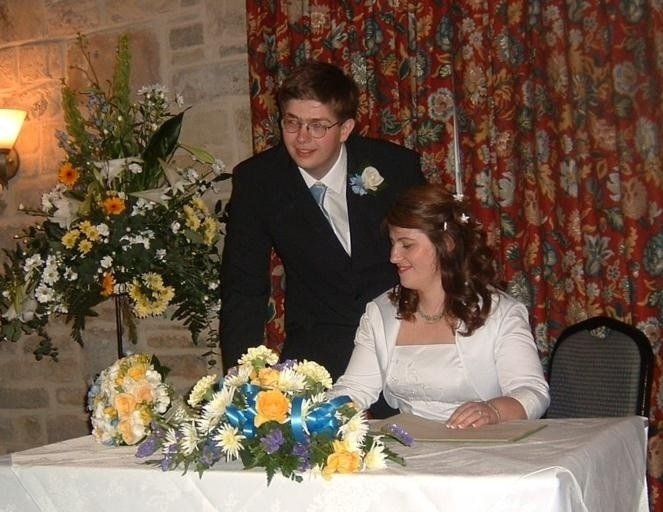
top-left (310, 182), bottom-right (348, 257)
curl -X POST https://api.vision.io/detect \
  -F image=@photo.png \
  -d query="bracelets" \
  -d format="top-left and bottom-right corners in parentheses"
top-left (483, 401), bottom-right (501, 424)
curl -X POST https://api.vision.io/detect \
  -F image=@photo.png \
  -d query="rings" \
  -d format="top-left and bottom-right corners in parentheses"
top-left (477, 410), bottom-right (482, 415)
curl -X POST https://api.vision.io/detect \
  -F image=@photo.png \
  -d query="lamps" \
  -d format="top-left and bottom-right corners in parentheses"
top-left (0, 109), bottom-right (28, 190)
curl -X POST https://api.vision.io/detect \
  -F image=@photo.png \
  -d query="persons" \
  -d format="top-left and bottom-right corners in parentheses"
top-left (336, 183), bottom-right (551, 430)
top-left (217, 61), bottom-right (429, 421)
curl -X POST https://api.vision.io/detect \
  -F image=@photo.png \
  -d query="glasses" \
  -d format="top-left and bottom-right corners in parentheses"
top-left (276, 112), bottom-right (352, 139)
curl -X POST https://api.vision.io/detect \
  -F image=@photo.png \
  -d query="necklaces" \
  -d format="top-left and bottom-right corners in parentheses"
top-left (416, 305), bottom-right (444, 321)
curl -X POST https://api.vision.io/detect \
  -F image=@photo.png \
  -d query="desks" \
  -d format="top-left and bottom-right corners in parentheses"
top-left (0, 415), bottom-right (650, 512)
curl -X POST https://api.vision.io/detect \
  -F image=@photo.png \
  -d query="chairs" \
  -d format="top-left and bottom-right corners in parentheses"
top-left (543, 316), bottom-right (654, 418)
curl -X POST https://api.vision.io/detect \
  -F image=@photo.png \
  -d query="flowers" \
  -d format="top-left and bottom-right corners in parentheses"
top-left (86, 350), bottom-right (176, 449)
top-left (349, 166), bottom-right (384, 196)
top-left (0, 33), bottom-right (232, 370)
top-left (135, 344), bottom-right (413, 487)
